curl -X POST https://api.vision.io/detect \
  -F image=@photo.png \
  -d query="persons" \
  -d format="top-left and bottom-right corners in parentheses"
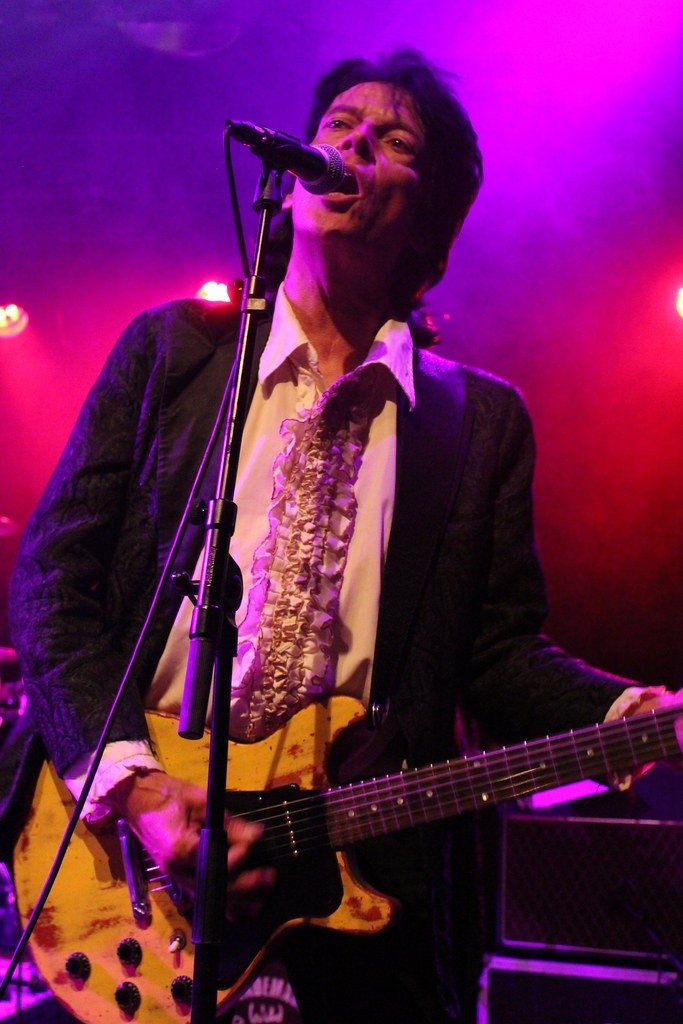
top-left (0, 44), bottom-right (683, 1024)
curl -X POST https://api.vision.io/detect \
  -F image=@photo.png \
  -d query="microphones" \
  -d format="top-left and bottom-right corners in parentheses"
top-left (223, 118), bottom-right (346, 196)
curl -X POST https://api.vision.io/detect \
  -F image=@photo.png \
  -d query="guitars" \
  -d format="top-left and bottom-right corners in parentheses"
top-left (12, 703), bottom-right (683, 1024)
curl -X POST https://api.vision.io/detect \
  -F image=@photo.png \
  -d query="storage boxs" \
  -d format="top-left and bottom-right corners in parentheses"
top-left (475, 948), bottom-right (683, 1024)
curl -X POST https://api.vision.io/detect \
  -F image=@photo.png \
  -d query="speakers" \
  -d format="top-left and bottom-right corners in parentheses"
top-left (496, 813), bottom-right (683, 961)
top-left (477, 955), bottom-right (683, 1024)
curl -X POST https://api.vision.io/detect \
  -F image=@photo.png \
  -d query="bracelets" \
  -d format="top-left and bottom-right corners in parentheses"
top-left (612, 685), bottom-right (675, 789)
